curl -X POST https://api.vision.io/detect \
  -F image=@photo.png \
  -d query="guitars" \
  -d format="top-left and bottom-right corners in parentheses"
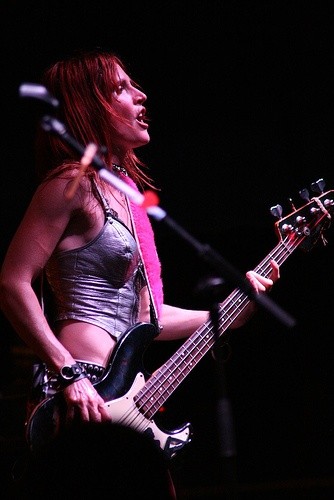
top-left (26, 178), bottom-right (334, 461)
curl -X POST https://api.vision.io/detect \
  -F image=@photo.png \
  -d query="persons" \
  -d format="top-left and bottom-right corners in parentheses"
top-left (0, 53), bottom-right (281, 500)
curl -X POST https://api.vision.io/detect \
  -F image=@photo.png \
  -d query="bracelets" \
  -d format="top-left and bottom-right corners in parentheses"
top-left (57, 363), bottom-right (89, 385)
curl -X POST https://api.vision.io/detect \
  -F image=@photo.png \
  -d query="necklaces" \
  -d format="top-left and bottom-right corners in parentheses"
top-left (112, 162), bottom-right (128, 176)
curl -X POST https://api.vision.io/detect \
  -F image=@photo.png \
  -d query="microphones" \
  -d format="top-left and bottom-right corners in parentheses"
top-left (18, 82), bottom-right (59, 113)
